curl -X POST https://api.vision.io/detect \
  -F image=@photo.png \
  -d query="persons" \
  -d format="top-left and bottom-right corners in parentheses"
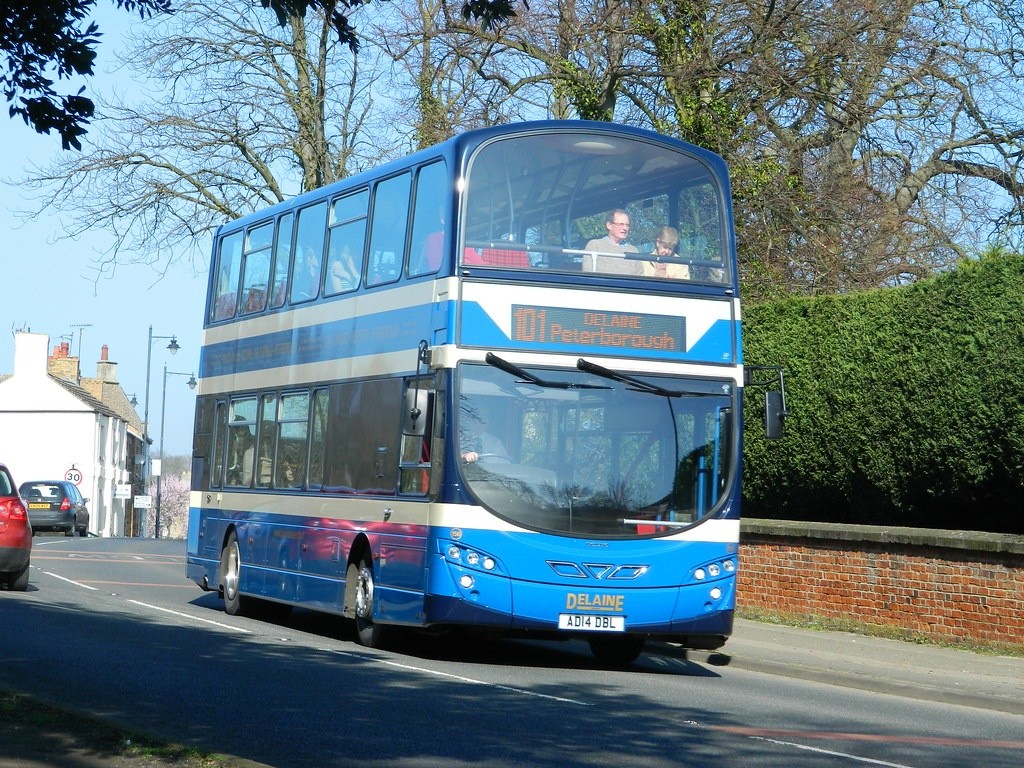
top-left (425, 214), bottom-right (485, 272)
top-left (582, 208), bottom-right (646, 277)
top-left (329, 242), bottom-right (360, 294)
top-left (460, 408), bottom-right (511, 463)
top-left (644, 227), bottom-right (691, 281)
top-left (291, 248), bottom-right (321, 301)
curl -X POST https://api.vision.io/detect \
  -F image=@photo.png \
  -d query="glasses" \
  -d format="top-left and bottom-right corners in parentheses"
top-left (610, 220), bottom-right (630, 227)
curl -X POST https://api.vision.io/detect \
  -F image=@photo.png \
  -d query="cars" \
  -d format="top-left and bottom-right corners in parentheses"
top-left (0, 463), bottom-right (33, 591)
top-left (17, 481), bottom-right (91, 537)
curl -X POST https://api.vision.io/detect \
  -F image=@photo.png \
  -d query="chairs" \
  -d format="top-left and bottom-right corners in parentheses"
top-left (427, 232), bottom-right (483, 269)
top-left (258, 456), bottom-right (272, 484)
top-left (216, 292), bottom-right (237, 321)
top-left (482, 248), bottom-right (528, 268)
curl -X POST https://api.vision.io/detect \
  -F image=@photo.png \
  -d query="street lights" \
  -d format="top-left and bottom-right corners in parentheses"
top-left (137, 324), bottom-right (181, 537)
top-left (154, 360), bottom-right (197, 537)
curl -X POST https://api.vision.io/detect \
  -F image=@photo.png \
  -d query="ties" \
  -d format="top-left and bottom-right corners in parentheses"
top-left (474, 438), bottom-right (483, 462)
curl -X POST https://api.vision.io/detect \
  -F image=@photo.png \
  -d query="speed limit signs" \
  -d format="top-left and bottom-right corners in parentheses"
top-left (65, 469), bottom-right (83, 486)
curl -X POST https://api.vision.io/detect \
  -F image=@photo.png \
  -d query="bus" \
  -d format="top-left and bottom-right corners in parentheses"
top-left (185, 118), bottom-right (791, 663)
